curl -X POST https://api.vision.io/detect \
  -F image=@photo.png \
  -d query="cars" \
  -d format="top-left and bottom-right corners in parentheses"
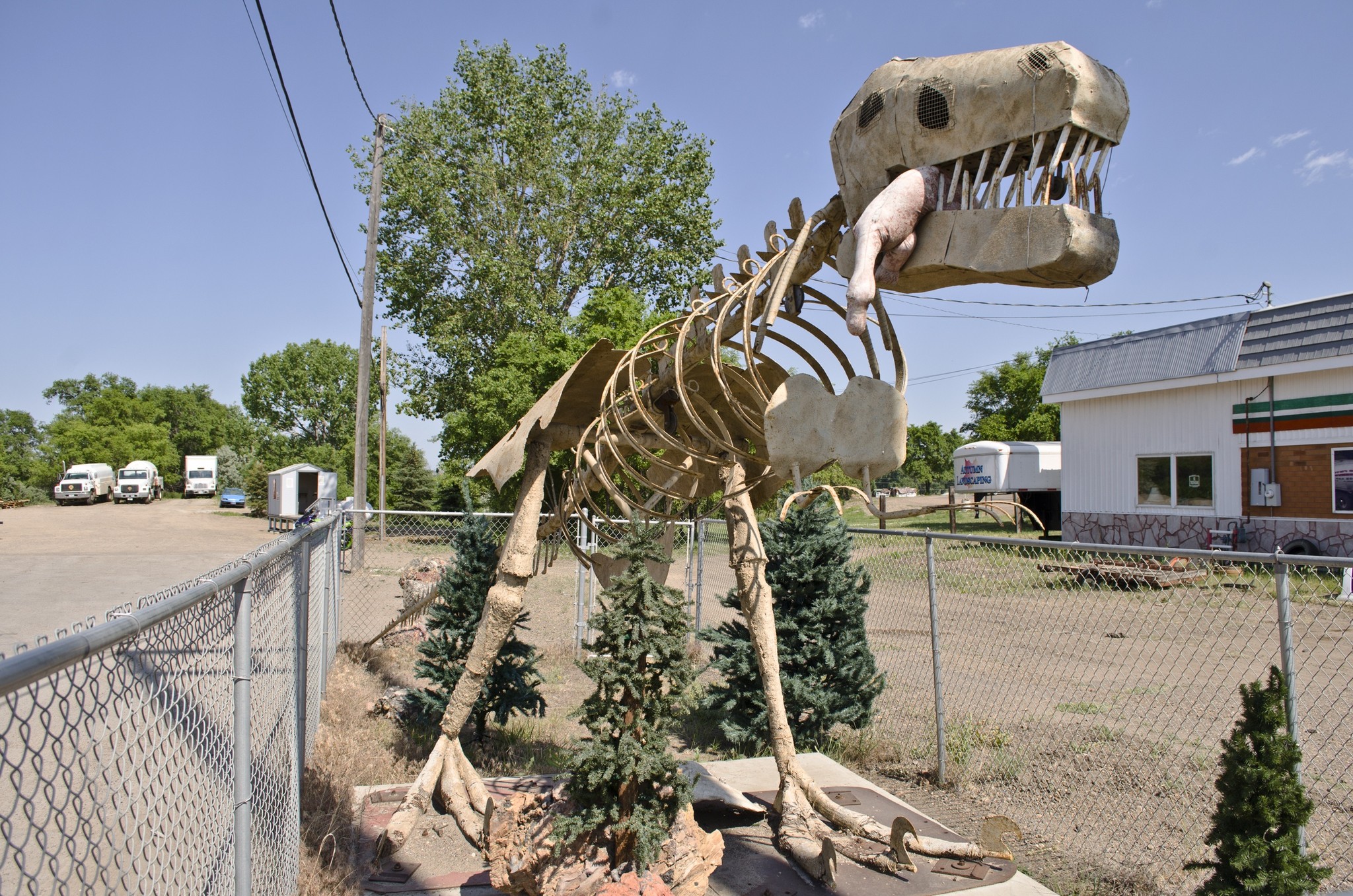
top-left (219, 487), bottom-right (247, 508)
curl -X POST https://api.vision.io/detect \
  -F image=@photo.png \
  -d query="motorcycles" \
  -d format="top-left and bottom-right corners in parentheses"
top-left (294, 497), bottom-right (353, 551)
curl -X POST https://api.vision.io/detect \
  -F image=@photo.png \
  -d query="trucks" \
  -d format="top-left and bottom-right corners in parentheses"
top-left (952, 440), bottom-right (1061, 531)
top-left (113, 460), bottom-right (164, 505)
top-left (54, 460), bottom-right (116, 506)
top-left (183, 455), bottom-right (219, 498)
